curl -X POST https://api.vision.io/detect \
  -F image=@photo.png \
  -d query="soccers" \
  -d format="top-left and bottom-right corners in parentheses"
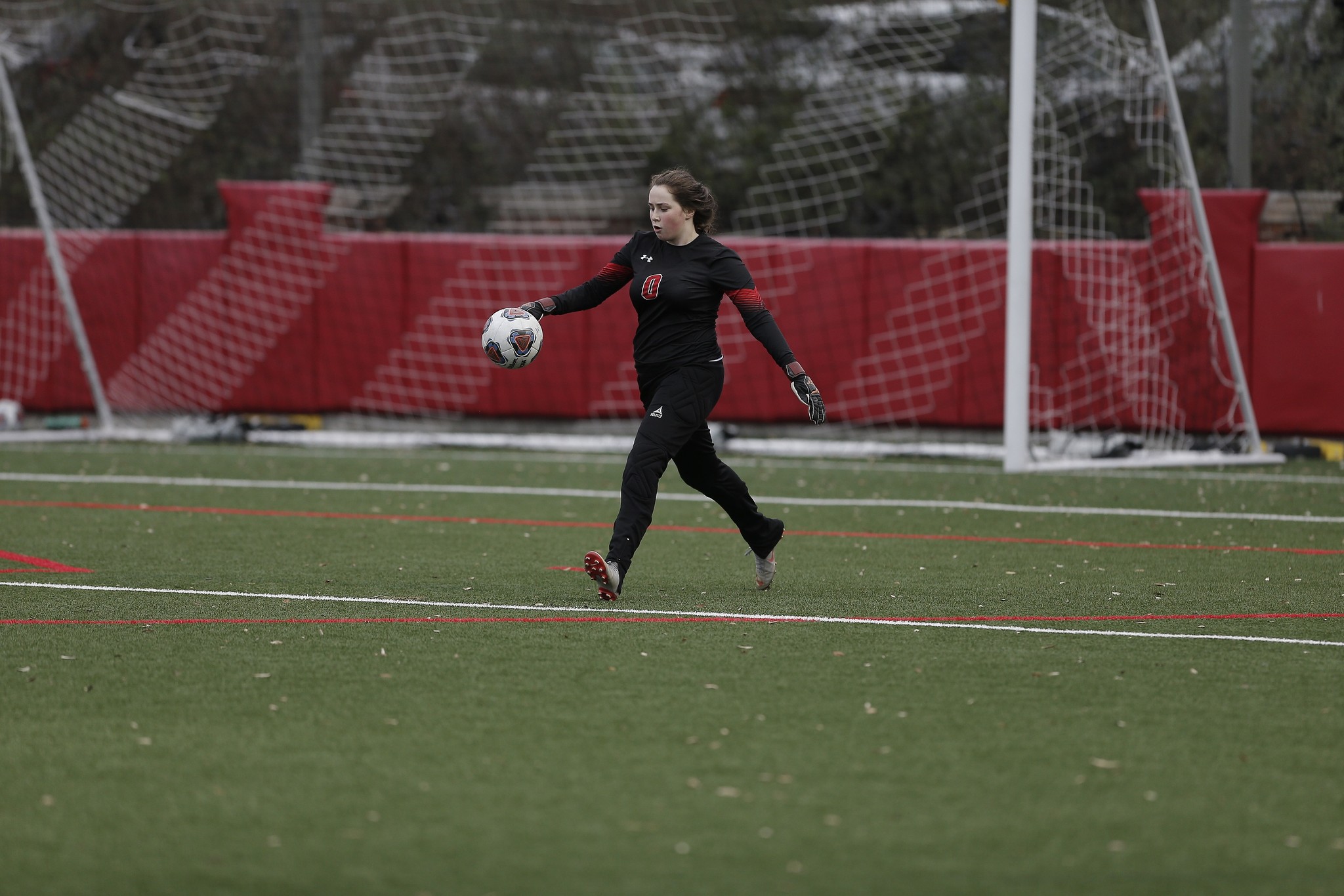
top-left (482, 308), bottom-right (544, 370)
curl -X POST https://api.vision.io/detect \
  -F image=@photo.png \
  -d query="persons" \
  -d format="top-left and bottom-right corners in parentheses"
top-left (518, 168), bottom-right (826, 600)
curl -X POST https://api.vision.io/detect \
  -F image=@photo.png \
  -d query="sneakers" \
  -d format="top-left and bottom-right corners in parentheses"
top-left (745, 528), bottom-right (786, 590)
top-left (584, 551), bottom-right (626, 601)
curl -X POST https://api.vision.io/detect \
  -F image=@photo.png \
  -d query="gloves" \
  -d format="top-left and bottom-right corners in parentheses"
top-left (517, 297), bottom-right (556, 323)
top-left (783, 362), bottom-right (825, 426)
